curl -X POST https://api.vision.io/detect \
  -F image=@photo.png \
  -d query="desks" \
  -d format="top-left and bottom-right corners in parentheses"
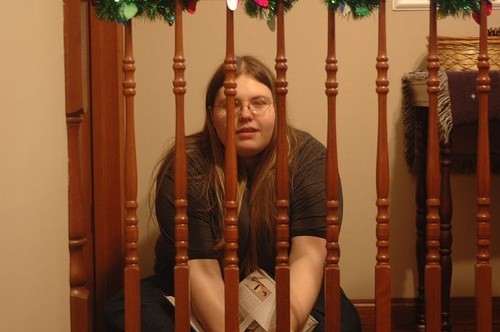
top-left (403, 71), bottom-right (500, 332)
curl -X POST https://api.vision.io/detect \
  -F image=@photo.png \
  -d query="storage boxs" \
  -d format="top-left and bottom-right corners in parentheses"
top-left (426, 33), bottom-right (500, 72)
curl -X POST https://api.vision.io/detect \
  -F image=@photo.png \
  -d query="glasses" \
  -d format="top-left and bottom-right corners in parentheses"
top-left (212, 95), bottom-right (274, 115)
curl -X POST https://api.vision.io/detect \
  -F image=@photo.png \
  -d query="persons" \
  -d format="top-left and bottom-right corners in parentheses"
top-left (104, 54), bottom-right (364, 332)
